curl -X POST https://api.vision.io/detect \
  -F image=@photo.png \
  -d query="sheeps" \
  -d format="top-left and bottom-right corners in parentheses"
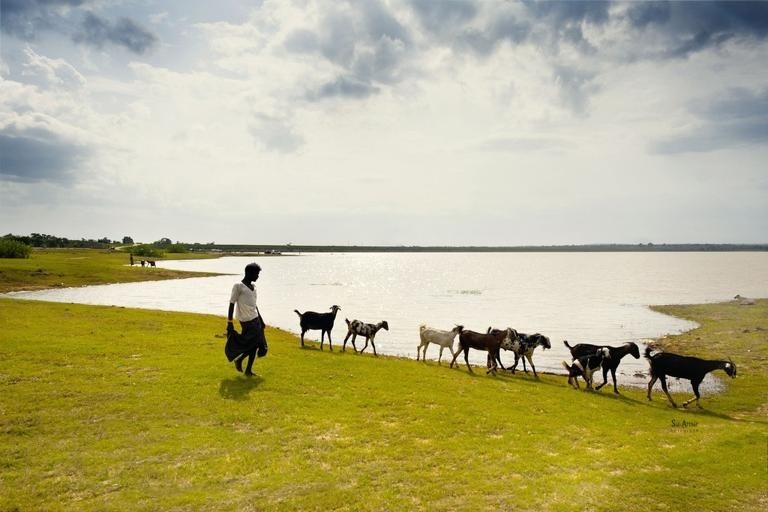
top-left (343, 317), bottom-right (389, 358)
top-left (416, 322), bottom-right (465, 368)
top-left (564, 339), bottom-right (641, 395)
top-left (561, 347), bottom-right (612, 391)
top-left (644, 345), bottom-right (737, 409)
top-left (141, 259), bottom-right (145, 267)
top-left (294, 305), bottom-right (341, 351)
top-left (449, 326), bottom-right (519, 376)
top-left (147, 259), bottom-right (156, 268)
top-left (485, 326), bottom-right (551, 377)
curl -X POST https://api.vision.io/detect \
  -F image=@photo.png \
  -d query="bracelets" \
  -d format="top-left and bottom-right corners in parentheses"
top-left (227, 320), bottom-right (233, 323)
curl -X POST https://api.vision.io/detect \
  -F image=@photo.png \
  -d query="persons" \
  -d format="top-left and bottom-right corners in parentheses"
top-left (226, 263), bottom-right (268, 377)
top-left (129, 253), bottom-right (134, 267)
